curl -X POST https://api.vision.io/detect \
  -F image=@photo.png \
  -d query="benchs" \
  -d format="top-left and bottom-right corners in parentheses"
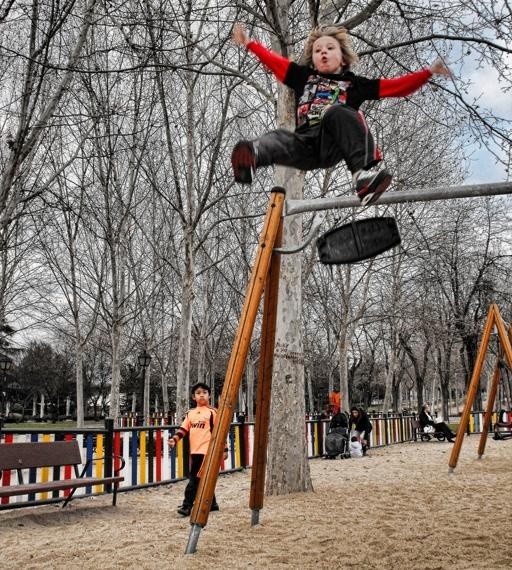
top-left (410, 421), bottom-right (445, 441)
top-left (0, 441), bottom-right (126, 513)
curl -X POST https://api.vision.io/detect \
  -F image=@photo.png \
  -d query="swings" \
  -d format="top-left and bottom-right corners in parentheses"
top-left (492, 335), bottom-right (512, 440)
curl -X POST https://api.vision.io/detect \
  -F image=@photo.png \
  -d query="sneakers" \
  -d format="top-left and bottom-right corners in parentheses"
top-left (231, 142), bottom-right (256, 185)
top-left (356, 169), bottom-right (390, 207)
top-left (451, 434), bottom-right (456, 438)
top-left (449, 439), bottom-right (454, 442)
top-left (177, 508), bottom-right (190, 516)
top-left (208, 504), bottom-right (219, 511)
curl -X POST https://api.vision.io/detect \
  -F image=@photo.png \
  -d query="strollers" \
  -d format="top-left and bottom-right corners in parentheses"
top-left (322, 410), bottom-right (351, 475)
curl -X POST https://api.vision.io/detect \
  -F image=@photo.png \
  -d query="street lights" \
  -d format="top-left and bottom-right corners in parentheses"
top-left (137, 350), bottom-right (151, 425)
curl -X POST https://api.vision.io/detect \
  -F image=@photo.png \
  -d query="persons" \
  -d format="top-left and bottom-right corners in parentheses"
top-left (168, 384), bottom-right (228, 517)
top-left (349, 437), bottom-right (364, 459)
top-left (230, 23), bottom-right (451, 206)
top-left (348, 406), bottom-right (372, 456)
top-left (419, 402), bottom-right (456, 443)
